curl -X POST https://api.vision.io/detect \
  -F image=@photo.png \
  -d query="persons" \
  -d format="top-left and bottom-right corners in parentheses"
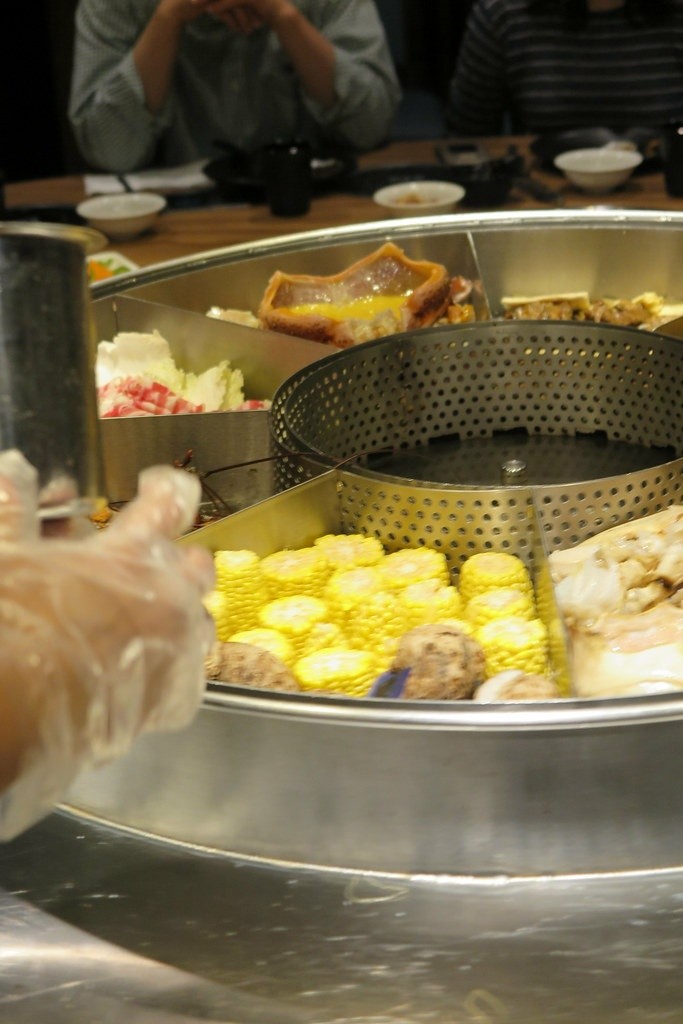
top-left (441, 0), bottom-right (683, 140)
top-left (0, 447), bottom-right (217, 796)
top-left (68, 0), bottom-right (403, 174)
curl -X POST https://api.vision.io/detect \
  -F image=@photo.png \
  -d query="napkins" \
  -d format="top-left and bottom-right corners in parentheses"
top-left (82, 159), bottom-right (210, 198)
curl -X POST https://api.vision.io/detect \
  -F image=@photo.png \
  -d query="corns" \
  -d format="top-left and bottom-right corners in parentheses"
top-left (204, 533), bottom-right (549, 699)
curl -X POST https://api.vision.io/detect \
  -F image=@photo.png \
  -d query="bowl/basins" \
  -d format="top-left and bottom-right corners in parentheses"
top-left (374, 180), bottom-right (466, 217)
top-left (76, 193), bottom-right (166, 238)
top-left (554, 148), bottom-right (643, 192)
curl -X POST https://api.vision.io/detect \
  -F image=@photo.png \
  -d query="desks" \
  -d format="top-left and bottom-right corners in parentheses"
top-left (3, 135), bottom-right (683, 269)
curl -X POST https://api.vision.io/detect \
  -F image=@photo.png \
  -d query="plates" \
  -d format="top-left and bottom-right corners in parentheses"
top-left (203, 143), bottom-right (357, 203)
top-left (530, 126), bottom-right (663, 176)
top-left (0, 221), bottom-right (108, 256)
top-left (360, 164), bottom-right (512, 208)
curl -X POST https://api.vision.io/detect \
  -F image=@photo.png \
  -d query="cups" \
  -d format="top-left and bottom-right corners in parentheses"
top-left (263, 139), bottom-right (312, 218)
top-left (665, 123), bottom-right (683, 197)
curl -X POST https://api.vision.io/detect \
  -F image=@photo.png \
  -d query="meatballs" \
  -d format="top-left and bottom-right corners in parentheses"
top-left (216, 642), bottom-right (304, 693)
top-left (394, 625), bottom-right (487, 699)
